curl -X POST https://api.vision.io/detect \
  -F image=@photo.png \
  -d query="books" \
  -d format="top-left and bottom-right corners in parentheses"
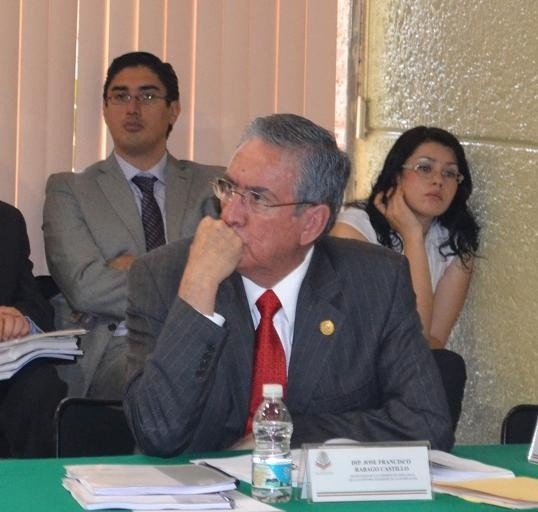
top-left (432, 476), bottom-right (538, 510)
top-left (1, 329), bottom-right (89, 380)
top-left (61, 464), bottom-right (237, 510)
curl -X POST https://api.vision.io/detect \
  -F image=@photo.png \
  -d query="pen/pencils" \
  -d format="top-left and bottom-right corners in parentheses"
top-left (199, 461), bottom-right (240, 486)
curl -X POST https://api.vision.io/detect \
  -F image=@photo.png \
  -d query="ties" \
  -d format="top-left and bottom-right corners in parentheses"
top-left (246, 290), bottom-right (287, 434)
top-left (131, 176), bottom-right (166, 252)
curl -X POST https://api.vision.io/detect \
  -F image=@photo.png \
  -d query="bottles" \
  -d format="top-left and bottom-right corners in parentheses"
top-left (252, 384), bottom-right (293, 504)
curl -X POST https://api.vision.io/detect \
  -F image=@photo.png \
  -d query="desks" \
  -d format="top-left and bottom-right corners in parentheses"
top-left (0, 442), bottom-right (537, 512)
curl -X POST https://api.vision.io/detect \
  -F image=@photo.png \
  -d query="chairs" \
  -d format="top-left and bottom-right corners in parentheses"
top-left (35, 274), bottom-right (60, 300)
top-left (52, 397), bottom-right (138, 457)
top-left (501, 404), bottom-right (538, 445)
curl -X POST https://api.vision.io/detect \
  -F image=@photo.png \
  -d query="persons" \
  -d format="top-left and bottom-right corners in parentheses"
top-left (41, 52), bottom-right (229, 398)
top-left (332, 125), bottom-right (480, 434)
top-left (0, 201), bottom-right (71, 457)
top-left (122, 114), bottom-right (453, 456)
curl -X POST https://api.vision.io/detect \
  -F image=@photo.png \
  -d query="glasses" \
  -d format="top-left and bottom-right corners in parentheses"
top-left (210, 177), bottom-right (318, 207)
top-left (400, 162), bottom-right (464, 184)
top-left (106, 93), bottom-right (166, 105)
